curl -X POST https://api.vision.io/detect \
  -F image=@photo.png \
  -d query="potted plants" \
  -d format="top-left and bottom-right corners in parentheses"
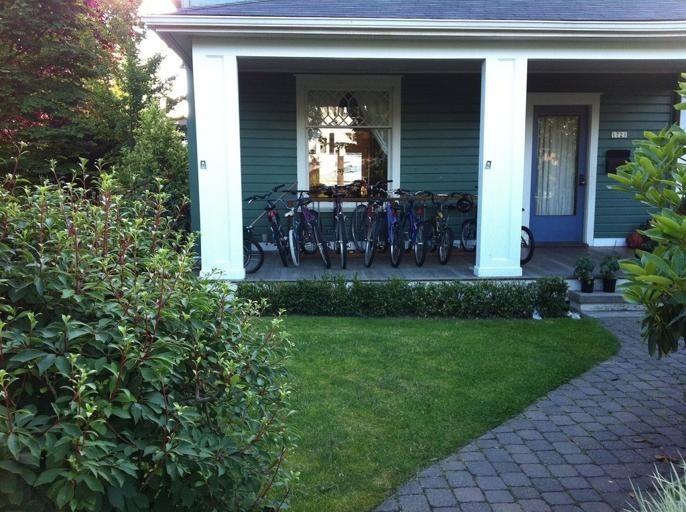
top-left (575, 250), bottom-right (621, 294)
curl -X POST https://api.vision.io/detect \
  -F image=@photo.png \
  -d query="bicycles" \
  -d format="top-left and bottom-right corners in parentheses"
top-left (242, 177), bottom-right (535, 274)
